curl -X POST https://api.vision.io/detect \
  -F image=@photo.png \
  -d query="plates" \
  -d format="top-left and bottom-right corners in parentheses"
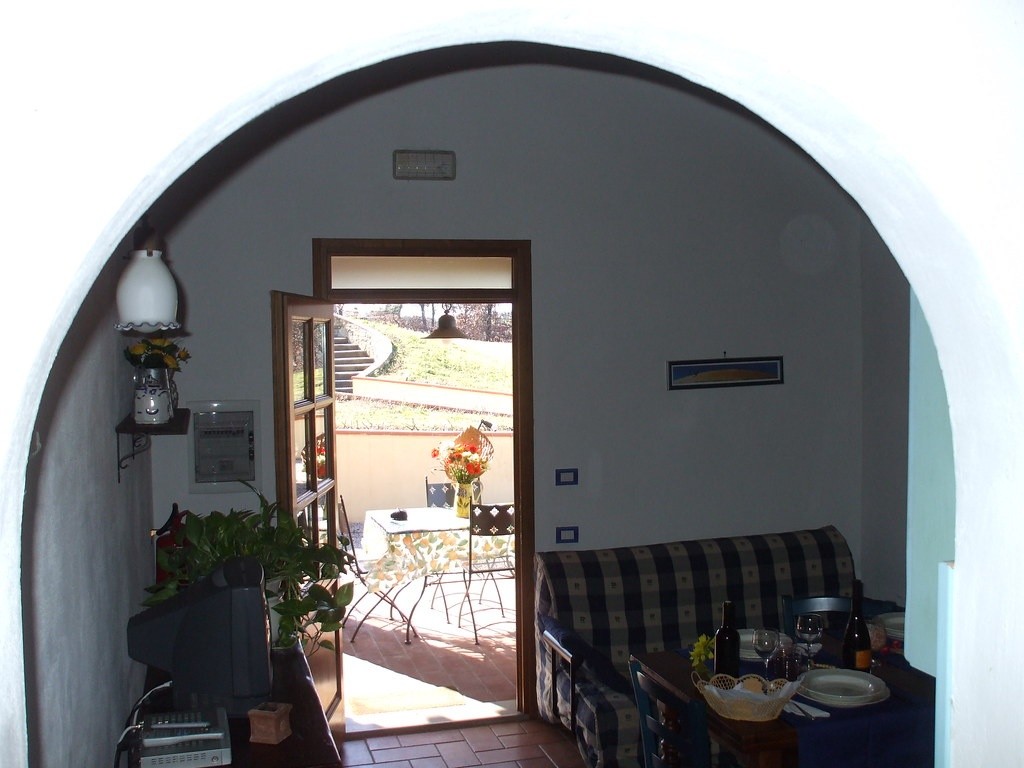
top-left (113, 322), bottom-right (181, 332)
top-left (879, 612), bottom-right (904, 640)
top-left (738, 628), bottom-right (792, 661)
top-left (794, 668), bottom-right (889, 706)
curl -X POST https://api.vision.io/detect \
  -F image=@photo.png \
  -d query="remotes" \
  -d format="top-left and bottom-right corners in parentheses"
top-left (150, 711), bottom-right (211, 729)
top-left (143, 728), bottom-right (225, 746)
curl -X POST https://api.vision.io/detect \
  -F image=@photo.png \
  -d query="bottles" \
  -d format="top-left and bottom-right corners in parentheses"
top-left (843, 579), bottom-right (871, 673)
top-left (715, 601), bottom-right (739, 677)
top-left (116, 249), bottom-right (178, 325)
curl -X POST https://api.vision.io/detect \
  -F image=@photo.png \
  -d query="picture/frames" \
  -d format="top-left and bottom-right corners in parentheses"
top-left (665, 355), bottom-right (785, 392)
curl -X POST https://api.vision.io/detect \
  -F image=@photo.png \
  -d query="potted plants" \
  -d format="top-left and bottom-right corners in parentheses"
top-left (139, 478), bottom-right (368, 653)
top-left (247, 690), bottom-right (295, 744)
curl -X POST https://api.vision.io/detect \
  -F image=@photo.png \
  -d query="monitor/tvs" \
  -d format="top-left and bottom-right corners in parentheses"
top-left (126, 556), bottom-right (276, 716)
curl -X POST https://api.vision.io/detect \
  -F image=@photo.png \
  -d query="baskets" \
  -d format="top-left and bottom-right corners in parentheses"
top-left (691, 671), bottom-right (796, 722)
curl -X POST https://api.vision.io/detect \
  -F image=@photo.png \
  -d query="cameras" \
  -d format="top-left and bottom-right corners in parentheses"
top-left (391, 508), bottom-right (407, 520)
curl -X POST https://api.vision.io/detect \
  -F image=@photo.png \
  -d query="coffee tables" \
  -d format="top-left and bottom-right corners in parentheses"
top-left (620, 625), bottom-right (936, 768)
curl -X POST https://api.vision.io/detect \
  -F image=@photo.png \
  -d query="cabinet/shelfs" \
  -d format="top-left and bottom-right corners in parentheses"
top-left (113, 330), bottom-right (191, 483)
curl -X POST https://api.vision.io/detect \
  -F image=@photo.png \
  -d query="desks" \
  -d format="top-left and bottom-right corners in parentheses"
top-left (128, 634), bottom-right (341, 768)
top-left (359, 505), bottom-right (515, 642)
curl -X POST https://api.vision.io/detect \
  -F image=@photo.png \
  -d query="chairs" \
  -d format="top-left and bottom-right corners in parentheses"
top-left (458, 497), bottom-right (516, 646)
top-left (425, 472), bottom-right (511, 624)
top-left (337, 495), bottom-right (427, 645)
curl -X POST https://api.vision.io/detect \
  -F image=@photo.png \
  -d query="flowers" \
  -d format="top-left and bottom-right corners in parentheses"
top-left (688, 630), bottom-right (714, 675)
top-left (430, 437), bottom-right (493, 482)
top-left (122, 330), bottom-right (191, 372)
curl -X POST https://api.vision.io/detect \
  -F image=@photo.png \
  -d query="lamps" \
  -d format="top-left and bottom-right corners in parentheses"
top-left (420, 302), bottom-right (470, 344)
top-left (391, 148), bottom-right (457, 182)
top-left (111, 215), bottom-right (182, 336)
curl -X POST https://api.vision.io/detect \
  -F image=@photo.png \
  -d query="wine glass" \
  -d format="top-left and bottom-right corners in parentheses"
top-left (753, 629), bottom-right (779, 681)
top-left (796, 613), bottom-right (824, 671)
top-left (777, 635), bottom-right (796, 679)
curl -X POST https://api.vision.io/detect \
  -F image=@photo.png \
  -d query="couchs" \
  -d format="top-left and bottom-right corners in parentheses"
top-left (530, 522), bottom-right (905, 768)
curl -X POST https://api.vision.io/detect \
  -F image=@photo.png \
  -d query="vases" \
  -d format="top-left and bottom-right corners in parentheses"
top-left (171, 380), bottom-right (178, 409)
top-left (132, 368), bottom-right (174, 425)
top-left (456, 480), bottom-right (477, 518)
